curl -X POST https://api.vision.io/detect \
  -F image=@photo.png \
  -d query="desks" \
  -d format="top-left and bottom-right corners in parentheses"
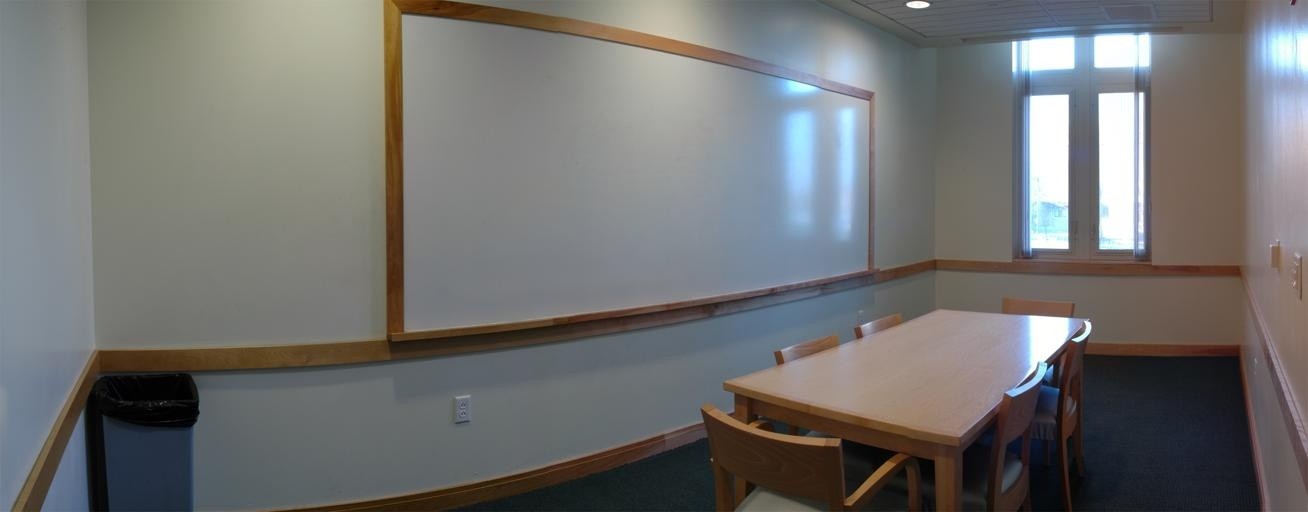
top-left (719, 307), bottom-right (1091, 510)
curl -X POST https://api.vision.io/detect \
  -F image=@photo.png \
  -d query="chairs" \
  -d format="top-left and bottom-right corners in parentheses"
top-left (773, 334), bottom-right (842, 435)
top-left (855, 312), bottom-right (904, 340)
top-left (701, 400), bottom-right (923, 512)
top-left (961, 359), bottom-right (1050, 511)
top-left (1032, 317), bottom-right (1092, 511)
top-left (999, 297), bottom-right (1078, 388)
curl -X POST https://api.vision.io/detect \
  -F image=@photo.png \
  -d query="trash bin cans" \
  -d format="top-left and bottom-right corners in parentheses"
top-left (93, 371), bottom-right (199, 512)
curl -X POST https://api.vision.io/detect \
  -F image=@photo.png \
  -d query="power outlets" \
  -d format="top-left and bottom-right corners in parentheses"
top-left (454, 394), bottom-right (471, 425)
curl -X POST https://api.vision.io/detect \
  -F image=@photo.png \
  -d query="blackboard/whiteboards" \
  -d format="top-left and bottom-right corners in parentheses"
top-left (383, 0), bottom-right (875, 341)
